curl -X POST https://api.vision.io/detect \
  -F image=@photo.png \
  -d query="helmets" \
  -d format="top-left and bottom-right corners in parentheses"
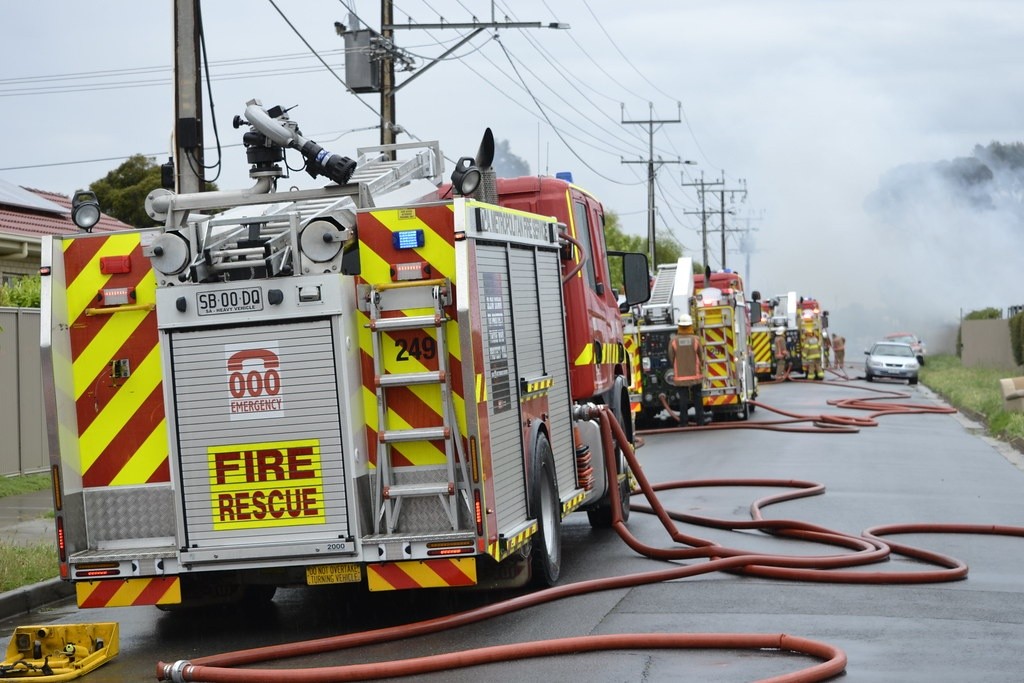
top-left (776, 326), bottom-right (785, 335)
top-left (678, 315), bottom-right (693, 326)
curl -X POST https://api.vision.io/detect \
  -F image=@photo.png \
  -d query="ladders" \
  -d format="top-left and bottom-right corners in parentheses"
top-left (642, 263), bottom-right (679, 316)
top-left (362, 280), bottom-right (464, 531)
top-left (698, 316), bottom-right (733, 390)
top-left (206, 136), bottom-right (444, 273)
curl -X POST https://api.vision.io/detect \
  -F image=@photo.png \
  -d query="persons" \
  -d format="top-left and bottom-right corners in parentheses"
top-left (821, 332), bottom-right (847, 370)
top-left (801, 329), bottom-right (825, 380)
top-left (774, 326), bottom-right (790, 381)
top-left (667, 312), bottom-right (705, 427)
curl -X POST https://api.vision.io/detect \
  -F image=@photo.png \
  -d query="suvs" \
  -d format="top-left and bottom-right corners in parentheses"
top-left (883, 333), bottom-right (926, 365)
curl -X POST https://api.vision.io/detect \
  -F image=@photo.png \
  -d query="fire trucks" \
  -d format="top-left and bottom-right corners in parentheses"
top-left (38, 94), bottom-right (650, 621)
top-left (749, 291), bottom-right (830, 379)
top-left (613, 256), bottom-right (760, 425)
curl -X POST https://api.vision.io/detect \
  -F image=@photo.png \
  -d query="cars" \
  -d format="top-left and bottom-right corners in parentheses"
top-left (864, 342), bottom-right (920, 385)
